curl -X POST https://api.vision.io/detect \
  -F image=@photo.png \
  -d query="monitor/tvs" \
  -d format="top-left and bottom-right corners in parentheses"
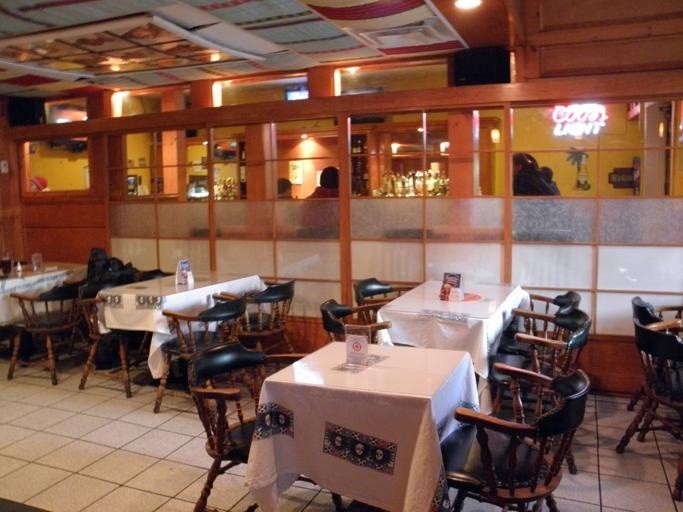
top-left (189, 175), bottom-right (210, 197)
top-left (334, 86), bottom-right (384, 126)
top-left (127, 174), bottom-right (138, 196)
top-left (284, 86), bottom-right (309, 101)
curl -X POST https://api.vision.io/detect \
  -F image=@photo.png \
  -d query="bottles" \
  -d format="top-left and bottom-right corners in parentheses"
top-left (380, 168), bottom-right (448, 197)
top-left (212, 177), bottom-right (246, 200)
top-left (15, 256), bottom-right (22, 273)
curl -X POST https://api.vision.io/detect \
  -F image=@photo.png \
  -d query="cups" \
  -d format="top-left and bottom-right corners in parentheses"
top-left (32, 252), bottom-right (43, 273)
top-left (0, 256), bottom-right (12, 276)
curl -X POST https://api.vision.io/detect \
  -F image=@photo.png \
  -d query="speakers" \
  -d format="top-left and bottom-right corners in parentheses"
top-left (8, 96), bottom-right (41, 126)
top-left (454, 46), bottom-right (507, 86)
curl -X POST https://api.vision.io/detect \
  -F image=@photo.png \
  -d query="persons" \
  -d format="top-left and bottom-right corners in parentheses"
top-left (513, 153), bottom-right (575, 242)
top-left (299, 166), bottom-right (339, 230)
top-left (28, 177), bottom-right (49, 193)
top-left (539, 165), bottom-right (561, 195)
top-left (278, 178), bottom-right (292, 198)
